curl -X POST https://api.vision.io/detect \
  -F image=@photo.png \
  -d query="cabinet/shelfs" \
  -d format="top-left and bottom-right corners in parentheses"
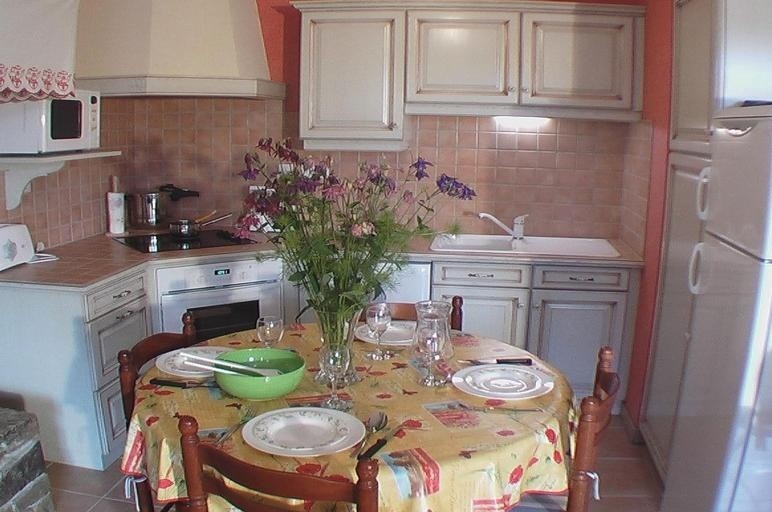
top-left (405, 0), bottom-right (646, 124)
top-left (289, 0), bottom-right (410, 151)
top-left (431, 261), bottom-right (642, 418)
top-left (0, 269), bottom-right (156, 472)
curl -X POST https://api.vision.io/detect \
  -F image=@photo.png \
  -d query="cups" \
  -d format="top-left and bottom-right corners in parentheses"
top-left (256, 317), bottom-right (283, 346)
top-left (107, 191), bottom-right (126, 234)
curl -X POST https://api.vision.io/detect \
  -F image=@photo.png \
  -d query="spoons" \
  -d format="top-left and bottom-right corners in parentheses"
top-left (351, 410), bottom-right (387, 459)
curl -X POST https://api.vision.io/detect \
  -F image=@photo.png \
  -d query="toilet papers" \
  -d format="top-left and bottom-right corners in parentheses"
top-left (104, 188), bottom-right (127, 236)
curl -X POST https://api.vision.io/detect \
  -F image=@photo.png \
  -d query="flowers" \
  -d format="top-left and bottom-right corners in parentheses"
top-left (230, 136), bottom-right (476, 343)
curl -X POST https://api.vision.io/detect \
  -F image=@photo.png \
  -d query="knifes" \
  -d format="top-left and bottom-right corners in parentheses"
top-left (456, 358), bottom-right (533, 365)
top-left (359, 423), bottom-right (405, 460)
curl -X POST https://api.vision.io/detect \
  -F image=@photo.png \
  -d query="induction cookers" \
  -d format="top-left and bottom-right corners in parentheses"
top-left (113, 229), bottom-right (260, 253)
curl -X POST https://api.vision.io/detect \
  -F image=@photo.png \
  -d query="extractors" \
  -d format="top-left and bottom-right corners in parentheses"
top-left (72, 0), bottom-right (286, 100)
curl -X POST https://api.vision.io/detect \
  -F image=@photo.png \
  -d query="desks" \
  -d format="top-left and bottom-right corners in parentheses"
top-left (131, 320), bottom-right (574, 511)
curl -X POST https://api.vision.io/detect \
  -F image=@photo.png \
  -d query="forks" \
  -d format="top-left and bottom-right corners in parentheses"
top-left (214, 407), bottom-right (256, 446)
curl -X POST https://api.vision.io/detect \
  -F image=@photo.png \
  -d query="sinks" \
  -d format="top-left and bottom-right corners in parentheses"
top-left (516, 232), bottom-right (622, 258)
top-left (431, 229), bottom-right (515, 254)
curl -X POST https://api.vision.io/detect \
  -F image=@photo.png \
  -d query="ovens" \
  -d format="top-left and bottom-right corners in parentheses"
top-left (155, 258), bottom-right (284, 342)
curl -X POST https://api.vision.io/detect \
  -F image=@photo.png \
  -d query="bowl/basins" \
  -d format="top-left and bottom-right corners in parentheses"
top-left (216, 348), bottom-right (305, 402)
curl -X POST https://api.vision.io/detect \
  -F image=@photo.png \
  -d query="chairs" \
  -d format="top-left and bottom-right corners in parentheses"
top-left (514, 345), bottom-right (618, 511)
top-left (177, 409), bottom-right (380, 512)
top-left (118, 331), bottom-right (184, 434)
top-left (182, 299), bottom-right (265, 342)
top-left (358, 292), bottom-right (463, 330)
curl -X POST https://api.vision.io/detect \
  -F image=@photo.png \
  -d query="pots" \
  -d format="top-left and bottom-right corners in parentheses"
top-left (169, 212), bottom-right (232, 237)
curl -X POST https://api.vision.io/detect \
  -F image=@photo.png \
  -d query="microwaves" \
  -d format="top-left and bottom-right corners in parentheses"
top-left (0, 89), bottom-right (100, 156)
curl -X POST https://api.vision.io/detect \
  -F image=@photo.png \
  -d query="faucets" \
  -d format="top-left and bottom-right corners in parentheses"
top-left (476, 210), bottom-right (528, 239)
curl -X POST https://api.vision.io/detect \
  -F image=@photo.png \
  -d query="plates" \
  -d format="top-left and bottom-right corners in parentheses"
top-left (355, 322), bottom-right (418, 345)
top-left (156, 346), bottom-right (232, 378)
top-left (241, 407), bottom-right (367, 458)
top-left (451, 365), bottom-right (555, 400)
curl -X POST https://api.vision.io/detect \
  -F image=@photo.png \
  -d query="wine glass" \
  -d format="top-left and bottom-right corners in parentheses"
top-left (318, 343), bottom-right (351, 410)
top-left (413, 300), bottom-right (453, 386)
top-left (365, 305), bottom-right (394, 361)
